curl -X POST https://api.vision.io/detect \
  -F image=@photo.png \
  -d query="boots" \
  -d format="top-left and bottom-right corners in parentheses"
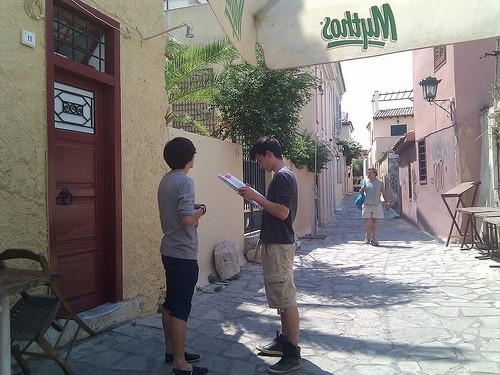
top-left (254, 330), bottom-right (288, 355)
top-left (267, 341), bottom-right (302, 375)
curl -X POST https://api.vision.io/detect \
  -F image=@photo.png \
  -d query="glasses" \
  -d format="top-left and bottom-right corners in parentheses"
top-left (256, 156), bottom-right (263, 165)
top-left (193, 154), bottom-right (196, 157)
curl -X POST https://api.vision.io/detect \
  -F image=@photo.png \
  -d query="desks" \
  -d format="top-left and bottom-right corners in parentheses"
top-left (456, 206), bottom-right (500, 268)
top-left (0, 268), bottom-right (62, 375)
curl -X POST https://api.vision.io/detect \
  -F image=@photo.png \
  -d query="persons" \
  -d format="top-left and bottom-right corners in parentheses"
top-left (238, 136), bottom-right (301, 374)
top-left (358, 167), bottom-right (391, 245)
top-left (157, 136), bottom-right (210, 375)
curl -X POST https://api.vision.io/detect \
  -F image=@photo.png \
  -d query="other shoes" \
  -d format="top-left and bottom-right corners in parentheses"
top-left (370, 239), bottom-right (378, 246)
top-left (363, 238), bottom-right (370, 244)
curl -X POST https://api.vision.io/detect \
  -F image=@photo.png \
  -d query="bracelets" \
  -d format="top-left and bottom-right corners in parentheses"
top-left (386, 203), bottom-right (390, 205)
top-left (200, 206), bottom-right (205, 215)
top-left (200, 204), bottom-right (206, 212)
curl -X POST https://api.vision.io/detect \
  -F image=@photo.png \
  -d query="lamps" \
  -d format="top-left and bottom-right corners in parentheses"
top-left (144, 19), bottom-right (194, 40)
top-left (419, 76), bottom-right (453, 120)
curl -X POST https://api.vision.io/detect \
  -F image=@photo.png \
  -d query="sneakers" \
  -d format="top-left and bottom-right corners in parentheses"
top-left (164, 351), bottom-right (201, 364)
top-left (172, 364), bottom-right (209, 375)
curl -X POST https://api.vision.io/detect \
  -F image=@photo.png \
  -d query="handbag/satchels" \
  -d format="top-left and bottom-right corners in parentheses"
top-left (355, 179), bottom-right (366, 210)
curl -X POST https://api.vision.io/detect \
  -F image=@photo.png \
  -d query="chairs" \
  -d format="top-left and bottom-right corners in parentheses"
top-left (0, 248), bottom-right (96, 375)
